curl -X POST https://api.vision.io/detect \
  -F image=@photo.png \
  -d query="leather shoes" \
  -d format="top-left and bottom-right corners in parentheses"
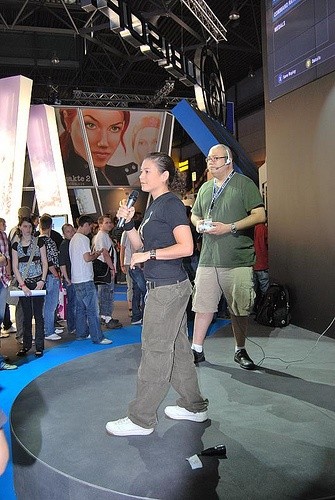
top-left (34, 351), bottom-right (43, 358)
top-left (2, 354), bottom-right (10, 361)
top-left (99, 338), bottom-right (112, 345)
top-left (1, 363), bottom-right (18, 370)
top-left (234, 349), bottom-right (254, 369)
top-left (16, 348), bottom-right (30, 356)
top-left (192, 349), bottom-right (205, 363)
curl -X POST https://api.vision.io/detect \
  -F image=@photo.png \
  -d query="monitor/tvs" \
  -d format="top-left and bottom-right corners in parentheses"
top-left (36, 215), bottom-right (69, 239)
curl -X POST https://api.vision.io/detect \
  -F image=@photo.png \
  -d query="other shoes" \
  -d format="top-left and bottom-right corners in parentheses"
top-left (1, 326), bottom-right (17, 333)
top-left (101, 318), bottom-right (122, 329)
top-left (0, 333), bottom-right (9, 338)
top-left (54, 317), bottom-right (67, 328)
top-left (67, 328), bottom-right (91, 341)
top-left (45, 333), bottom-right (61, 341)
top-left (128, 310), bottom-right (143, 325)
top-left (55, 329), bottom-right (63, 334)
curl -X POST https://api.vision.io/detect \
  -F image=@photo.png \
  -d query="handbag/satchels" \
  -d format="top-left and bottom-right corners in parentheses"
top-left (6, 283), bottom-right (21, 306)
top-left (92, 258), bottom-right (112, 285)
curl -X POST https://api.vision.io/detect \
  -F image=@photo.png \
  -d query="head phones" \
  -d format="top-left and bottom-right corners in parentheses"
top-left (219, 144), bottom-right (231, 165)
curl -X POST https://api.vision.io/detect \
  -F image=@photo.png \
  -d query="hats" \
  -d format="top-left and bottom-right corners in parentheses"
top-left (18, 206), bottom-right (34, 218)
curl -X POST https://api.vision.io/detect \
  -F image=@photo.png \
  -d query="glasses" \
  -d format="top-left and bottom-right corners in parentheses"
top-left (205, 155), bottom-right (226, 163)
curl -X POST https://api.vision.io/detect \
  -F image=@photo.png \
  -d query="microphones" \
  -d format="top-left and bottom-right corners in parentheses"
top-left (116, 190), bottom-right (139, 229)
top-left (216, 164), bottom-right (225, 170)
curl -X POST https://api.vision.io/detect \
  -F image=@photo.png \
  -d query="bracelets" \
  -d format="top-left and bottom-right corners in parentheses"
top-left (40, 280), bottom-right (45, 283)
top-left (123, 220), bottom-right (133, 231)
top-left (150, 249), bottom-right (156, 261)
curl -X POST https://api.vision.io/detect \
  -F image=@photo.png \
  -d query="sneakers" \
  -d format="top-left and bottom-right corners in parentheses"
top-left (164, 405), bottom-right (209, 423)
top-left (106, 417), bottom-right (154, 437)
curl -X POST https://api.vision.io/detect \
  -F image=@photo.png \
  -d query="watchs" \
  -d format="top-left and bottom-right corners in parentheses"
top-left (231, 223), bottom-right (237, 234)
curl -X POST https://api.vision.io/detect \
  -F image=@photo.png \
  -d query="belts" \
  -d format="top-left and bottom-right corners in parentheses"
top-left (146, 275), bottom-right (188, 290)
top-left (24, 277), bottom-right (42, 283)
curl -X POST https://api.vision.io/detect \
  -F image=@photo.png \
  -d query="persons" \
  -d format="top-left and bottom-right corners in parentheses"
top-left (105, 151), bottom-right (211, 436)
top-left (0, 201), bottom-right (267, 474)
top-left (189, 144), bottom-right (267, 368)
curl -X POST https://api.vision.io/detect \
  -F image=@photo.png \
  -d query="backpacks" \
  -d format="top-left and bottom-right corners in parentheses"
top-left (253, 282), bottom-right (291, 327)
